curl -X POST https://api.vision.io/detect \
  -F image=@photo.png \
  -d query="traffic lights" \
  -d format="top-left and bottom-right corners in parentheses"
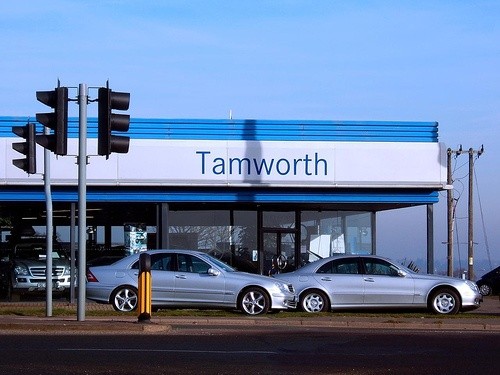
top-left (98, 80), bottom-right (130, 160)
top-left (35, 79), bottom-right (68, 160)
top-left (12, 119), bottom-right (36, 177)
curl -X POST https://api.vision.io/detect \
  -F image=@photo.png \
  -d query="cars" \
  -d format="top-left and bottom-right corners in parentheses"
top-left (476, 266), bottom-right (500, 296)
top-left (86, 249), bottom-right (299, 316)
top-left (273, 255), bottom-right (483, 315)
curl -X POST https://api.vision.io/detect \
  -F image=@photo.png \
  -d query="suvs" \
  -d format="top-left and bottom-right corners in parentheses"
top-left (6, 238), bottom-right (79, 302)
